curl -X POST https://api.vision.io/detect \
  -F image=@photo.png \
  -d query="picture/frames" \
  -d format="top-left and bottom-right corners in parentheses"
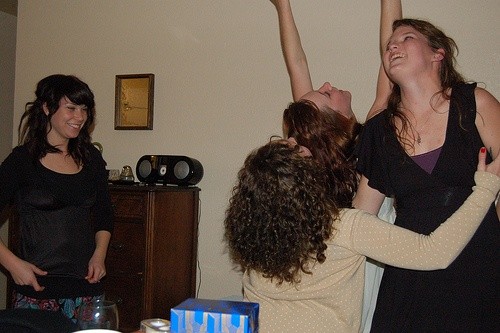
top-left (114, 73), bottom-right (154, 130)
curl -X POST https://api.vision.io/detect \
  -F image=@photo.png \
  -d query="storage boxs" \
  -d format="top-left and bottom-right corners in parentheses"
top-left (170, 299), bottom-right (259, 333)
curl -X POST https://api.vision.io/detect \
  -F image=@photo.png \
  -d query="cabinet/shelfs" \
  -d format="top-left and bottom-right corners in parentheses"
top-left (104, 185), bottom-right (200, 333)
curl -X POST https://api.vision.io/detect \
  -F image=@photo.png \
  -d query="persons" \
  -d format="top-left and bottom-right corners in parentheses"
top-left (1, 75), bottom-right (112, 330)
top-left (224, 136), bottom-right (500, 333)
top-left (270, 0), bottom-right (402, 333)
top-left (351, 18), bottom-right (500, 332)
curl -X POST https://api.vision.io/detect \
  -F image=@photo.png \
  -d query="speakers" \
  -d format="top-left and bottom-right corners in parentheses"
top-left (136, 154), bottom-right (204, 186)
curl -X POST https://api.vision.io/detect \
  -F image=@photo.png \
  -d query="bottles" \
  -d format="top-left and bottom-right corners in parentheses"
top-left (108, 169), bottom-right (119, 180)
top-left (120, 166), bottom-right (134, 182)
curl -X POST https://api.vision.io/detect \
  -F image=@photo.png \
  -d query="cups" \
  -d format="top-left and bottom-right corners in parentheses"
top-left (77, 300), bottom-right (119, 333)
